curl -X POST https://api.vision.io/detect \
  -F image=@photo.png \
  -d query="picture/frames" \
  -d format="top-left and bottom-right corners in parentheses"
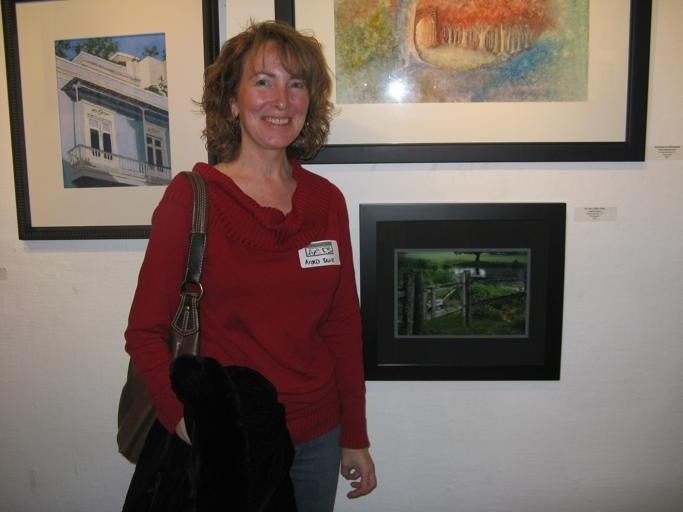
top-left (272, 1), bottom-right (652, 165)
top-left (357, 200), bottom-right (567, 384)
top-left (0, 0), bottom-right (221, 242)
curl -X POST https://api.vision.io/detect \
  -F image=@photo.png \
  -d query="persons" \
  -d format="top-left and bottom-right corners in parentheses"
top-left (120, 17), bottom-right (379, 512)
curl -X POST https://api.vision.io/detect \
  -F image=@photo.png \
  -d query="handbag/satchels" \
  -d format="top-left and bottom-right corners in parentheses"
top-left (113, 172), bottom-right (207, 462)
